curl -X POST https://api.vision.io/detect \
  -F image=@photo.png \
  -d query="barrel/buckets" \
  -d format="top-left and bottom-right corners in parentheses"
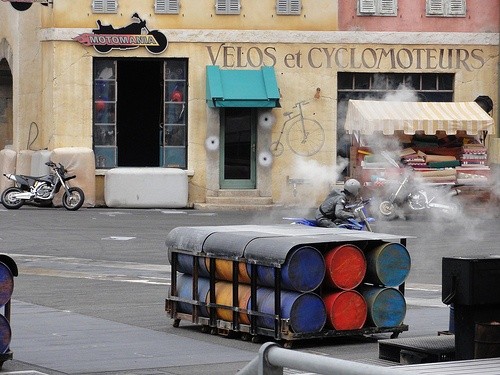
top-left (247, 287), bottom-right (327, 333)
top-left (206, 281), bottom-right (258, 325)
top-left (205, 257), bottom-right (252, 284)
top-left (366, 242), bottom-right (411, 286)
top-left (168, 248), bottom-right (211, 278)
top-left (168, 274), bottom-right (210, 317)
top-left (324, 244), bottom-right (366, 290)
top-left (356, 285), bottom-right (406, 328)
top-left (322, 290), bottom-right (368, 330)
top-left (246, 245), bottom-right (326, 293)
top-left (441, 254), bottom-right (500, 358)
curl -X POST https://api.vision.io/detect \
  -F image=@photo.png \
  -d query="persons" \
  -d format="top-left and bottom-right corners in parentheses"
top-left (316, 178), bottom-right (361, 228)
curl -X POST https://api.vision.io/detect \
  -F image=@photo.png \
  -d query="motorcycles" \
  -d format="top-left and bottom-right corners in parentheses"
top-left (1, 161), bottom-right (85, 210)
top-left (91, 12), bottom-right (168, 54)
top-left (378, 158), bottom-right (459, 222)
top-left (283, 196), bottom-right (377, 232)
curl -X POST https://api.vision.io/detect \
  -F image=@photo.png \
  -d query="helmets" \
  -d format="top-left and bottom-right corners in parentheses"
top-left (344, 178), bottom-right (361, 197)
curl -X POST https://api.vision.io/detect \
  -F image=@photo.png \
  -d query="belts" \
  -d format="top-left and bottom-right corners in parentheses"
top-left (318, 205), bottom-right (331, 218)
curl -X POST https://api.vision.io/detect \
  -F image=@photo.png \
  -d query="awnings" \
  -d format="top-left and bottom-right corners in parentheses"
top-left (205, 64), bottom-right (281, 110)
top-left (347, 98), bottom-right (494, 146)
